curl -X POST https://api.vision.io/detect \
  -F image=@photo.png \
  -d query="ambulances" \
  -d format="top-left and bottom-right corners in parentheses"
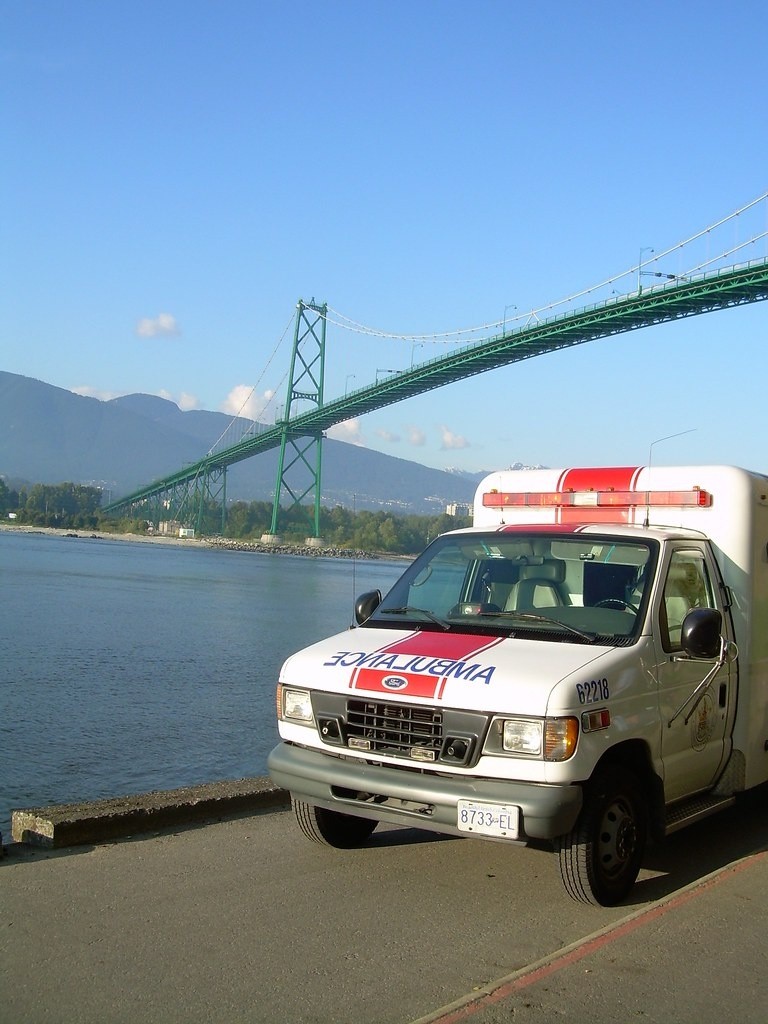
top-left (265, 463), bottom-right (768, 907)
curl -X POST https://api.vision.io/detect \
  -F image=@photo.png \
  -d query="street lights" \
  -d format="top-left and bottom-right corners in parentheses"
top-left (410, 344), bottom-right (423, 372)
top-left (502, 304), bottom-right (518, 338)
top-left (345, 374), bottom-right (355, 398)
top-left (637, 246), bottom-right (654, 296)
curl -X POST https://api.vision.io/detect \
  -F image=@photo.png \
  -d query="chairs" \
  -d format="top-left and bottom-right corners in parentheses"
top-left (504, 579), bottom-right (563, 612)
top-left (625, 580), bottom-right (691, 646)
